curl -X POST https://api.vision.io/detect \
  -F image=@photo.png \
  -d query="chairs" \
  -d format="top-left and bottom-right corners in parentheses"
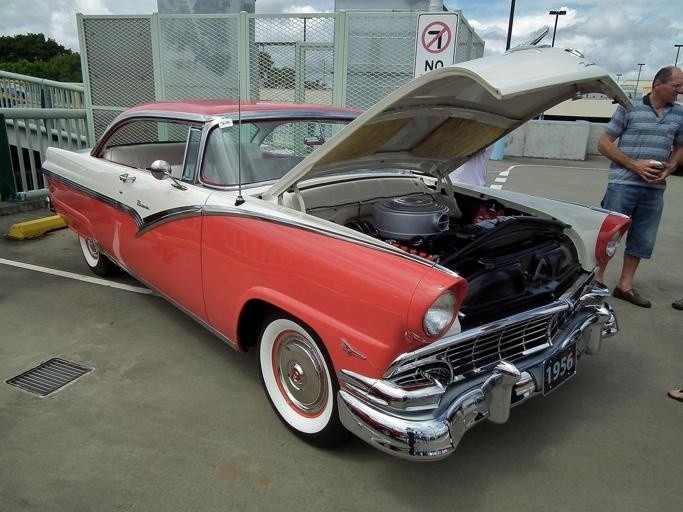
top-left (246, 157), bottom-right (308, 183)
top-left (170, 157), bottom-right (252, 185)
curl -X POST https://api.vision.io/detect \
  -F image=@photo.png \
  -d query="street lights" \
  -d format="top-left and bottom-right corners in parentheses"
top-left (615, 73), bottom-right (622, 84)
top-left (548, 10), bottom-right (566, 47)
top-left (634, 63), bottom-right (645, 98)
top-left (673, 44), bottom-right (683, 67)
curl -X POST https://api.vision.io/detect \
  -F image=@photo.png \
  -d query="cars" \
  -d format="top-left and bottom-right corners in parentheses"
top-left (39, 47), bottom-right (634, 465)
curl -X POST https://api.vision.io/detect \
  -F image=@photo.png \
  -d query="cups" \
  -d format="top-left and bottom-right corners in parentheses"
top-left (646, 160), bottom-right (663, 184)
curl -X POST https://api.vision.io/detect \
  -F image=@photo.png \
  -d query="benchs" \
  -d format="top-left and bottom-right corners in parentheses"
top-left (103, 139), bottom-right (260, 169)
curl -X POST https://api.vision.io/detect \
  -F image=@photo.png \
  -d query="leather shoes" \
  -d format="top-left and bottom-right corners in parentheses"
top-left (613, 286), bottom-right (651, 309)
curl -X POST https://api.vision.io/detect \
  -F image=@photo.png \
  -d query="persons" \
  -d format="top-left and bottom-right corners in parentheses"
top-left (668, 389), bottom-right (683, 402)
top-left (597, 66), bottom-right (683, 308)
